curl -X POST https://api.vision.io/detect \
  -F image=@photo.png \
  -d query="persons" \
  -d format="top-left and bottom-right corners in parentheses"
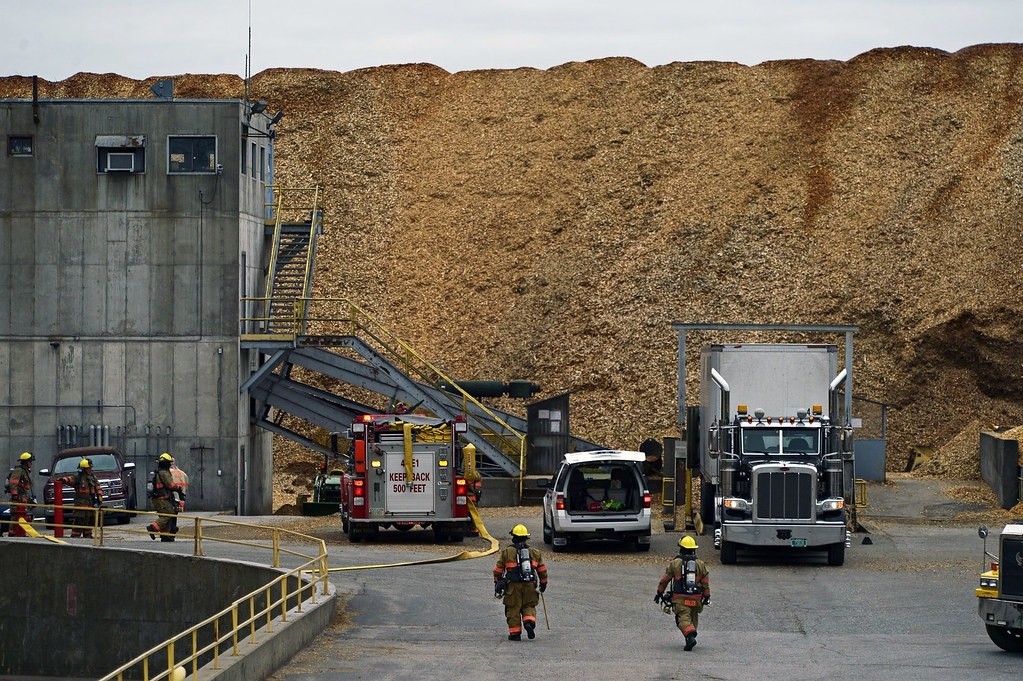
top-left (654, 536), bottom-right (711, 651)
top-left (8, 453), bottom-right (35, 537)
top-left (146, 453), bottom-right (180, 542)
top-left (492, 524), bottom-right (548, 641)
top-left (58, 459), bottom-right (102, 538)
top-left (169, 458), bottom-right (189, 512)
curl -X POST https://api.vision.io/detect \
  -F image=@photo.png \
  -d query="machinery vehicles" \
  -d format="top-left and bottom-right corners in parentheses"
top-left (975, 522), bottom-right (1023, 655)
top-left (686, 340), bottom-right (855, 568)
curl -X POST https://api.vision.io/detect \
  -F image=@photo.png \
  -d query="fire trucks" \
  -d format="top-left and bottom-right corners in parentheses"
top-left (328, 412), bottom-right (472, 544)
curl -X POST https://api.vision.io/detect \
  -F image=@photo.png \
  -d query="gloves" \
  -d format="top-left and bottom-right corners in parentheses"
top-left (97, 494), bottom-right (103, 507)
top-left (539, 583), bottom-right (546, 592)
top-left (11, 495), bottom-right (18, 501)
top-left (179, 490), bottom-right (186, 501)
top-left (495, 584), bottom-right (502, 593)
top-left (56, 478), bottom-right (66, 484)
top-left (703, 598), bottom-right (709, 605)
top-left (654, 590), bottom-right (664, 604)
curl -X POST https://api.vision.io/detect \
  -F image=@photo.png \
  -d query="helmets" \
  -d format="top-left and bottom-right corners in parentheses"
top-left (19, 452), bottom-right (36, 461)
top-left (677, 535), bottom-right (699, 549)
top-left (159, 452), bottom-right (173, 462)
top-left (511, 523), bottom-right (530, 536)
top-left (79, 459), bottom-right (94, 468)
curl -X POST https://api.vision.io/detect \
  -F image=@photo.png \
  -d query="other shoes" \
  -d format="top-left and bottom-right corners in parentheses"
top-left (83, 536), bottom-right (93, 538)
top-left (161, 538), bottom-right (174, 542)
top-left (147, 526), bottom-right (155, 540)
top-left (684, 637), bottom-right (697, 651)
top-left (507, 634), bottom-right (521, 641)
top-left (171, 527), bottom-right (179, 534)
top-left (524, 623), bottom-right (535, 639)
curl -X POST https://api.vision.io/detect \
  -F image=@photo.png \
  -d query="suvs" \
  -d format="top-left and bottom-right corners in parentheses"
top-left (38, 446), bottom-right (137, 530)
top-left (536, 448), bottom-right (652, 553)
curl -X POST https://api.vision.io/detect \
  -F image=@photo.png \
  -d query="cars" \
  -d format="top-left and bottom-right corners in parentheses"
top-left (0, 504), bottom-right (33, 538)
